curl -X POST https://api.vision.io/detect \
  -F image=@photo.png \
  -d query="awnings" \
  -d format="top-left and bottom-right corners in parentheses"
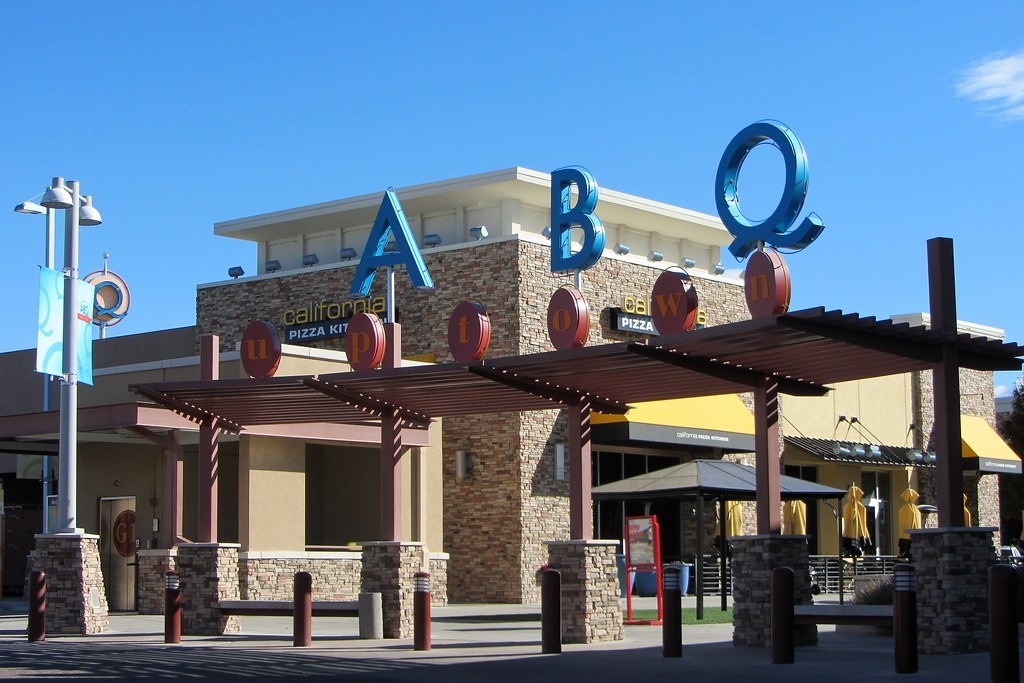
top-left (961, 415), bottom-right (1022, 473)
top-left (590, 393), bottom-right (755, 452)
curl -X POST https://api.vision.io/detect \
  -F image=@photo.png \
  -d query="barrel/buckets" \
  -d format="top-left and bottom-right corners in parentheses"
top-left (1001, 548), bottom-right (1011, 552)
top-left (635, 572), bottom-right (657, 597)
top-left (663, 565), bottom-right (689, 596)
top-left (616, 554), bottom-right (635, 597)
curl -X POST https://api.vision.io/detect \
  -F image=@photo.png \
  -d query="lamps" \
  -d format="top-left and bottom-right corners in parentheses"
top-left (228, 266), bottom-right (244, 279)
top-left (340, 248), bottom-right (357, 260)
top-left (264, 259), bottom-right (281, 273)
top-left (384, 241), bottom-right (399, 252)
top-left (423, 234), bottom-right (441, 247)
top-left (832, 442), bottom-right (936, 463)
top-left (613, 242), bottom-right (630, 255)
top-left (302, 254), bottom-right (319, 267)
top-left (648, 250), bottom-right (663, 262)
top-left (713, 265), bottom-right (725, 276)
top-left (469, 226), bottom-right (489, 241)
top-left (680, 257), bottom-right (696, 269)
top-left (541, 226), bottom-right (551, 240)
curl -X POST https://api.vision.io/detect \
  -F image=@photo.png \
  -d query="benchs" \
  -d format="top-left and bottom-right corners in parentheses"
top-left (794, 604), bottom-right (893, 626)
top-left (222, 599), bottom-right (359, 617)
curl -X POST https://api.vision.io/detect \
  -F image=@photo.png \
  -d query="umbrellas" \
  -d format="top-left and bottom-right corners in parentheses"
top-left (963, 493), bottom-right (971, 527)
top-left (715, 501), bottom-right (742, 537)
top-left (898, 487), bottom-right (922, 554)
top-left (782, 500), bottom-right (806, 535)
top-left (843, 485), bottom-right (873, 575)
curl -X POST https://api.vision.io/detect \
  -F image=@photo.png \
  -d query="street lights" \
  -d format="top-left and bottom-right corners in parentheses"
top-left (13, 199), bottom-right (57, 535)
top-left (41, 176), bottom-right (105, 536)
